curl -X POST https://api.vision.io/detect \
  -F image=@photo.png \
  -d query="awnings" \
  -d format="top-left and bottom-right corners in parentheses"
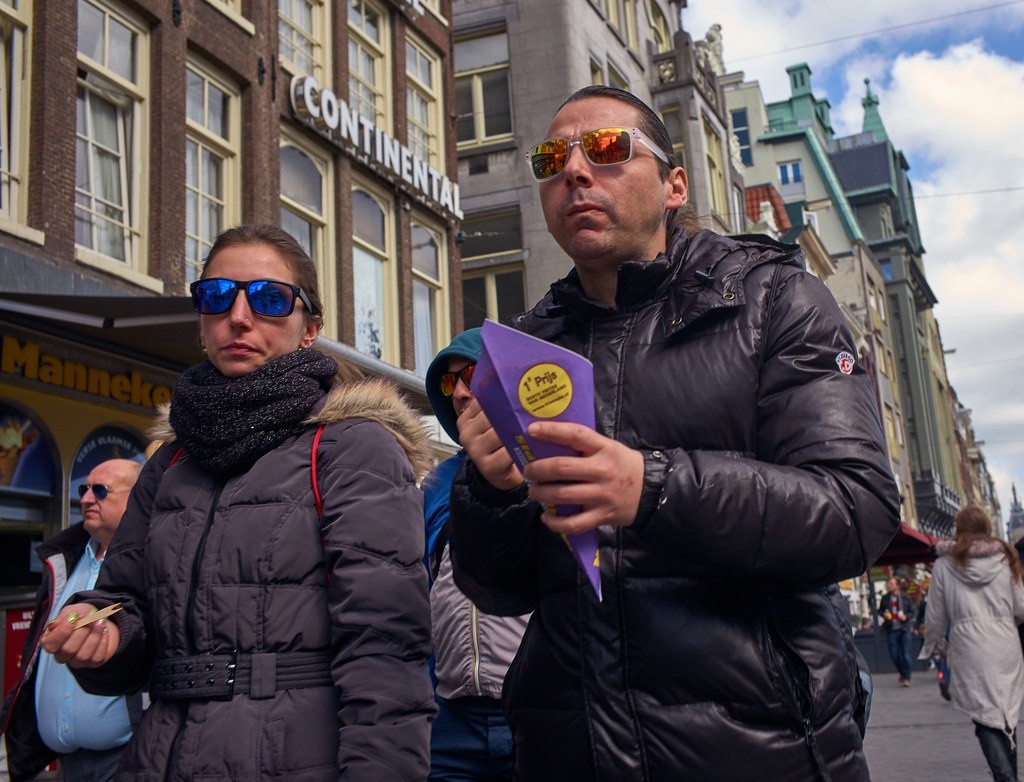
top-left (869, 522), bottom-right (938, 566)
top-left (1, 289), bottom-right (439, 416)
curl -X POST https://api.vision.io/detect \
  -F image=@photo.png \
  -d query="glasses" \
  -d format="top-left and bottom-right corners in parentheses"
top-left (189, 277), bottom-right (313, 318)
top-left (524, 125), bottom-right (670, 184)
top-left (440, 364), bottom-right (477, 397)
top-left (78, 484), bottom-right (113, 500)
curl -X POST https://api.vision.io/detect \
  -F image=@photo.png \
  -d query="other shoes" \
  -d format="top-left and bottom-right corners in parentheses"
top-left (899, 675), bottom-right (904, 683)
top-left (937, 682), bottom-right (951, 702)
top-left (903, 678), bottom-right (911, 686)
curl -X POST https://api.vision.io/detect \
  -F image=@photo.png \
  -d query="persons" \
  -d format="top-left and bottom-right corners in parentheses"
top-left (449, 84), bottom-right (901, 782)
top-left (40, 224), bottom-right (439, 782)
top-left (879, 574), bottom-right (915, 689)
top-left (0, 452), bottom-right (152, 782)
top-left (419, 326), bottom-right (537, 782)
top-left (914, 508), bottom-right (1024, 782)
